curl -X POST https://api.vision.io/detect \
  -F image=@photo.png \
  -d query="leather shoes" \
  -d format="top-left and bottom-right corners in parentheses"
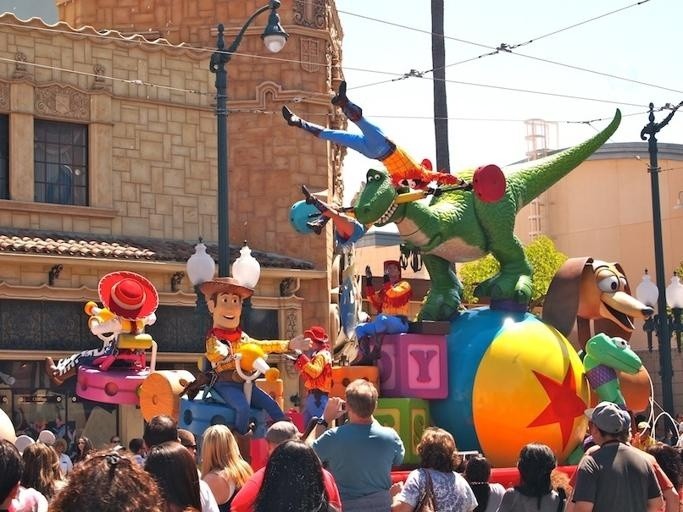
top-left (281, 105), bottom-right (300, 128)
top-left (306, 220), bottom-right (324, 232)
top-left (330, 80), bottom-right (347, 109)
top-left (302, 182), bottom-right (318, 205)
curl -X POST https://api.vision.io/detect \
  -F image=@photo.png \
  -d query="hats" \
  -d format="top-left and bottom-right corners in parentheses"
top-left (416, 159), bottom-right (434, 189)
top-left (304, 326), bottom-right (329, 343)
top-left (384, 261), bottom-right (401, 268)
top-left (264, 421), bottom-right (298, 443)
top-left (582, 400), bottom-right (625, 433)
top-left (637, 421), bottom-right (652, 429)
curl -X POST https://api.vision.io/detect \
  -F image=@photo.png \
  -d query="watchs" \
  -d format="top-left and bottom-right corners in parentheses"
top-left (313, 418), bottom-right (328, 428)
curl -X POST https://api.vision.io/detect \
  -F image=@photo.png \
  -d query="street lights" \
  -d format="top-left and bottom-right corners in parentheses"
top-left (635, 102), bottom-right (683, 443)
top-left (186, 1), bottom-right (289, 351)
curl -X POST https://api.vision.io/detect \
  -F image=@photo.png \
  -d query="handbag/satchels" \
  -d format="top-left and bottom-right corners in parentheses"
top-left (416, 466), bottom-right (437, 512)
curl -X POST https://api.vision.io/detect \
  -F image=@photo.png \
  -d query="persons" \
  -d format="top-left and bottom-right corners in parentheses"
top-left (0, 410), bottom-right (341, 512)
top-left (390, 424), bottom-right (566, 511)
top-left (566, 399), bottom-right (682, 512)
top-left (305, 379), bottom-right (405, 511)
top-left (282, 80), bottom-right (473, 195)
top-left (300, 185), bottom-right (374, 245)
top-left (350, 259), bottom-right (411, 366)
top-left (286, 327), bottom-right (332, 435)
top-left (43, 271), bottom-right (161, 385)
top-left (199, 277), bottom-right (312, 435)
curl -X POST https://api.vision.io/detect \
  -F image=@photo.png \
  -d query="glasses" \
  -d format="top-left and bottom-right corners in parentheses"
top-left (586, 418), bottom-right (599, 429)
top-left (185, 443), bottom-right (198, 450)
top-left (112, 440), bottom-right (121, 444)
